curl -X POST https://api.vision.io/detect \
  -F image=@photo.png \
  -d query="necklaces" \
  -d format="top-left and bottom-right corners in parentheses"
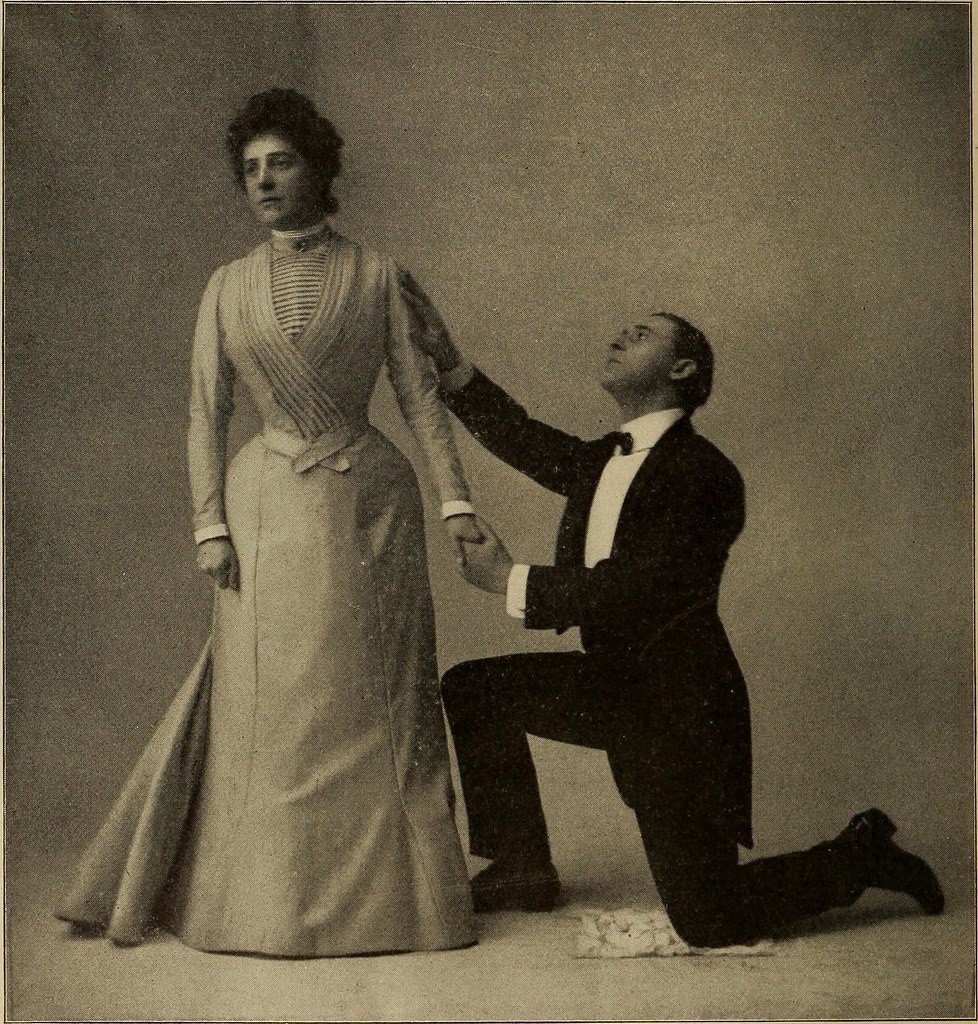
top-left (272, 219), bottom-right (327, 238)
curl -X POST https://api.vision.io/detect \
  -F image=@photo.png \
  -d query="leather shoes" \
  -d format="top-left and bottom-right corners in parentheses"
top-left (844, 807), bottom-right (945, 916)
top-left (470, 861), bottom-right (560, 912)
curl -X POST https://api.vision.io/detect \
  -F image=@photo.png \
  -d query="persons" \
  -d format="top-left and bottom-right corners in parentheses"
top-left (52, 88), bottom-right (481, 958)
top-left (400, 267), bottom-right (943, 949)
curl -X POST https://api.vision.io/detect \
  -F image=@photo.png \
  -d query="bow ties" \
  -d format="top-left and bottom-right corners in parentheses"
top-left (601, 432), bottom-right (634, 456)
